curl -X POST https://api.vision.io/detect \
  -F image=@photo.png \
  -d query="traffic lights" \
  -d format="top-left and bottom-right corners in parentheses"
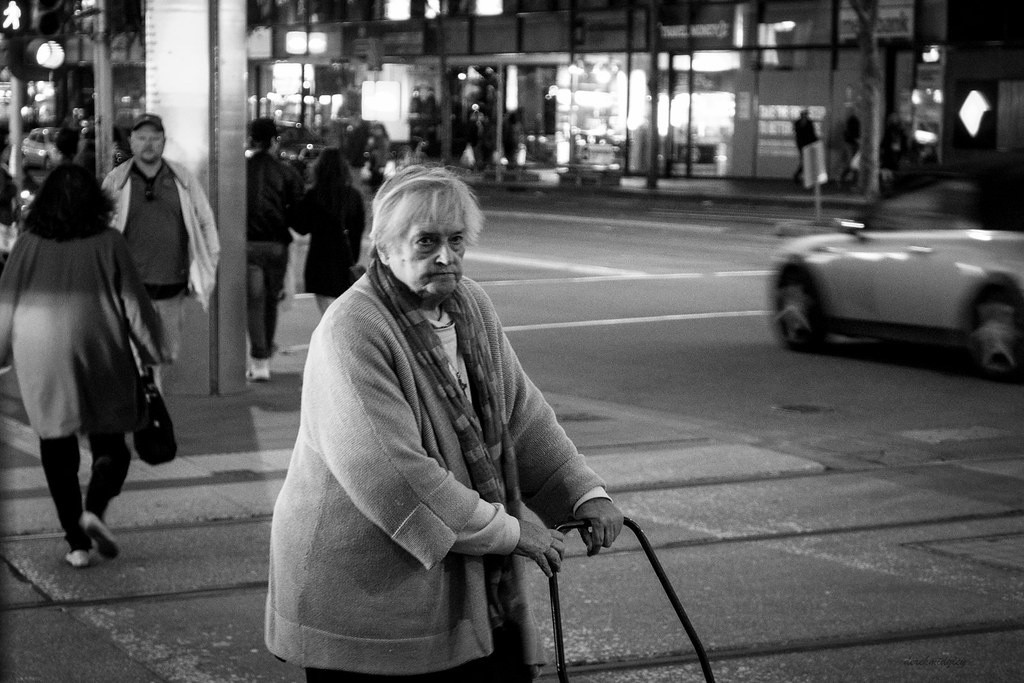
top-left (0, 0), bottom-right (65, 82)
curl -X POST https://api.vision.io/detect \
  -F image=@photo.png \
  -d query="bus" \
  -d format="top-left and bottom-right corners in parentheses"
top-left (53, 61), bottom-right (151, 131)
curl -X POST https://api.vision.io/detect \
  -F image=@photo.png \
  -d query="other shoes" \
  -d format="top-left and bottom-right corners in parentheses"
top-left (245, 356), bottom-right (273, 380)
top-left (78, 512), bottom-right (120, 559)
top-left (66, 549), bottom-right (89, 567)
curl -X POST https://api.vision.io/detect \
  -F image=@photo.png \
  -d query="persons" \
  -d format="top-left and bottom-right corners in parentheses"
top-left (791, 110), bottom-right (818, 186)
top-left (102, 113), bottom-right (221, 391)
top-left (0, 163), bottom-right (172, 567)
top-left (840, 108), bottom-right (861, 187)
top-left (247, 118), bottom-right (367, 381)
top-left (502, 106), bottom-right (527, 169)
top-left (266, 164), bottom-right (624, 683)
top-left (55, 117), bottom-right (96, 174)
top-left (0, 164), bottom-right (18, 265)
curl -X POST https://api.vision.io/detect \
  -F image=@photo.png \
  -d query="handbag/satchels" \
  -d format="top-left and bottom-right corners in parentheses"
top-left (134, 371), bottom-right (177, 465)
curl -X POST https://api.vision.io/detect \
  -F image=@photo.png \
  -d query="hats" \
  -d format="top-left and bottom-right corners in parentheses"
top-left (129, 114), bottom-right (163, 130)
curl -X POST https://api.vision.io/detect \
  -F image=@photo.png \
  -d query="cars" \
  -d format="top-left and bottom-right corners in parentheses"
top-left (21, 128), bottom-right (59, 172)
top-left (244, 118), bottom-right (327, 184)
top-left (767, 168), bottom-right (1024, 383)
